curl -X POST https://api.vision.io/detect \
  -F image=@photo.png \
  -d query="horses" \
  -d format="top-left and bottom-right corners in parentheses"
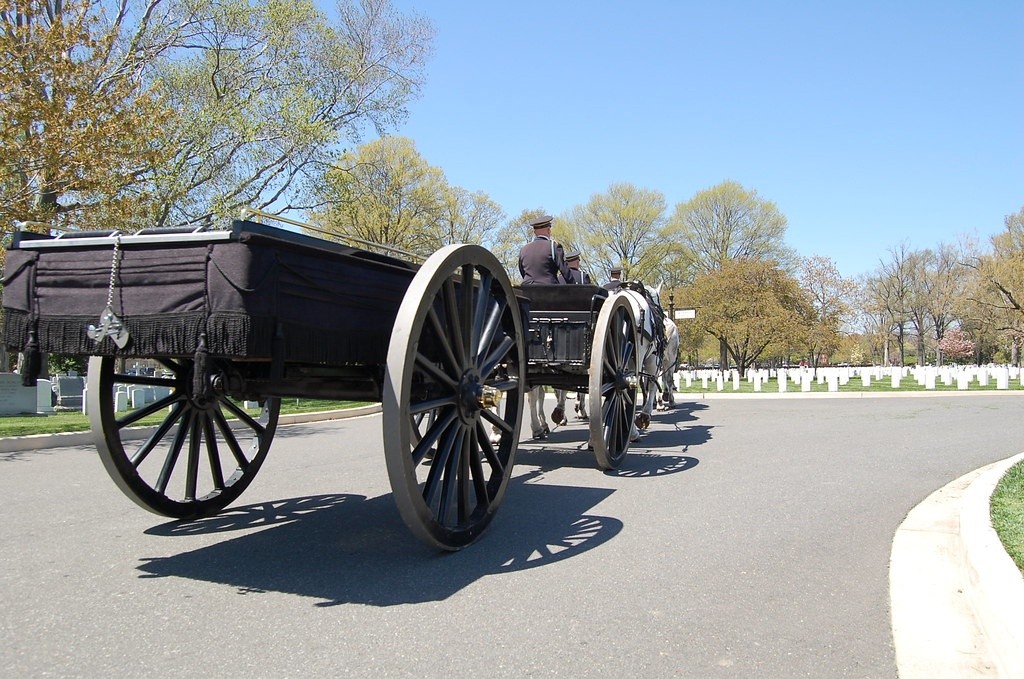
top-left (527, 279), bottom-right (679, 452)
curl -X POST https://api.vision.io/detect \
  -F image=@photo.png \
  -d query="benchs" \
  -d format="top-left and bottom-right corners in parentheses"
top-left (519, 283), bottom-right (607, 311)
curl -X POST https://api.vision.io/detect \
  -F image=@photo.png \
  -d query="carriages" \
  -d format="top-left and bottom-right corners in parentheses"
top-left (0, 204), bottom-right (684, 555)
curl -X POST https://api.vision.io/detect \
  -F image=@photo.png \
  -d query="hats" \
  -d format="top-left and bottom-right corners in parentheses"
top-left (566, 251), bottom-right (581, 261)
top-left (609, 267), bottom-right (623, 273)
top-left (530, 216), bottom-right (553, 229)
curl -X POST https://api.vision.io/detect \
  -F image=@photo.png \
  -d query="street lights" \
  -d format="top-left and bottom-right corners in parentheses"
top-left (666, 289), bottom-right (676, 321)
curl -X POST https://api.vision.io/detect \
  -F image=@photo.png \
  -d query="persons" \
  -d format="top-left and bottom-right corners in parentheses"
top-left (518, 216), bottom-right (579, 286)
top-left (558, 251), bottom-right (591, 285)
top-left (602, 267), bottom-right (623, 291)
